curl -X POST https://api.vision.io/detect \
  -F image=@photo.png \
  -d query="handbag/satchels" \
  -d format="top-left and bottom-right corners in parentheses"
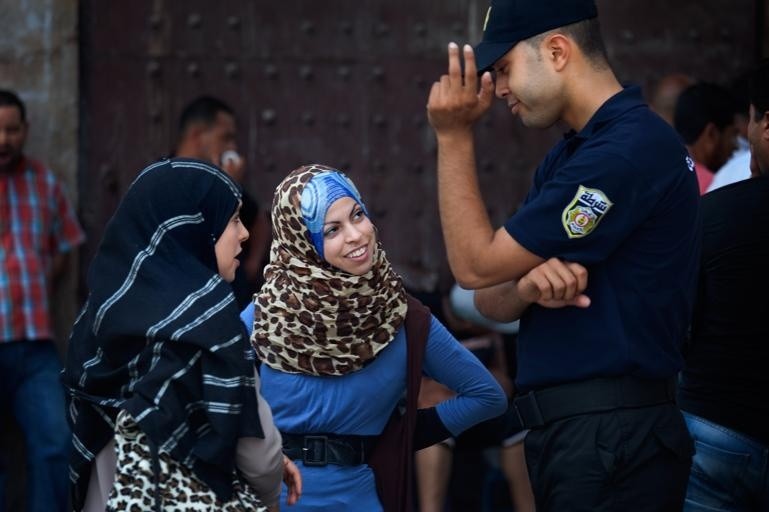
top-left (368, 293), bottom-right (431, 512)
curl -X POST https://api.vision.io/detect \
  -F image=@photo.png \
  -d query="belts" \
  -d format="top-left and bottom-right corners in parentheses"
top-left (506, 375), bottom-right (675, 432)
top-left (281, 433), bottom-right (375, 466)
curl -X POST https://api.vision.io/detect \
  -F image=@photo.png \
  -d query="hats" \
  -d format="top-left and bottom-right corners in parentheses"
top-left (457, 0), bottom-right (597, 77)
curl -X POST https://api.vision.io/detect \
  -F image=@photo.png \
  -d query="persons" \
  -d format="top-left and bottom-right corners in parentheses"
top-left (231, 160), bottom-right (509, 512)
top-left (674, 59), bottom-right (769, 512)
top-left (0, 87), bottom-right (86, 512)
top-left (161, 94), bottom-right (270, 309)
top-left (429, 1), bottom-right (701, 511)
top-left (55, 158), bottom-right (306, 512)
top-left (413, 251), bottom-right (536, 511)
